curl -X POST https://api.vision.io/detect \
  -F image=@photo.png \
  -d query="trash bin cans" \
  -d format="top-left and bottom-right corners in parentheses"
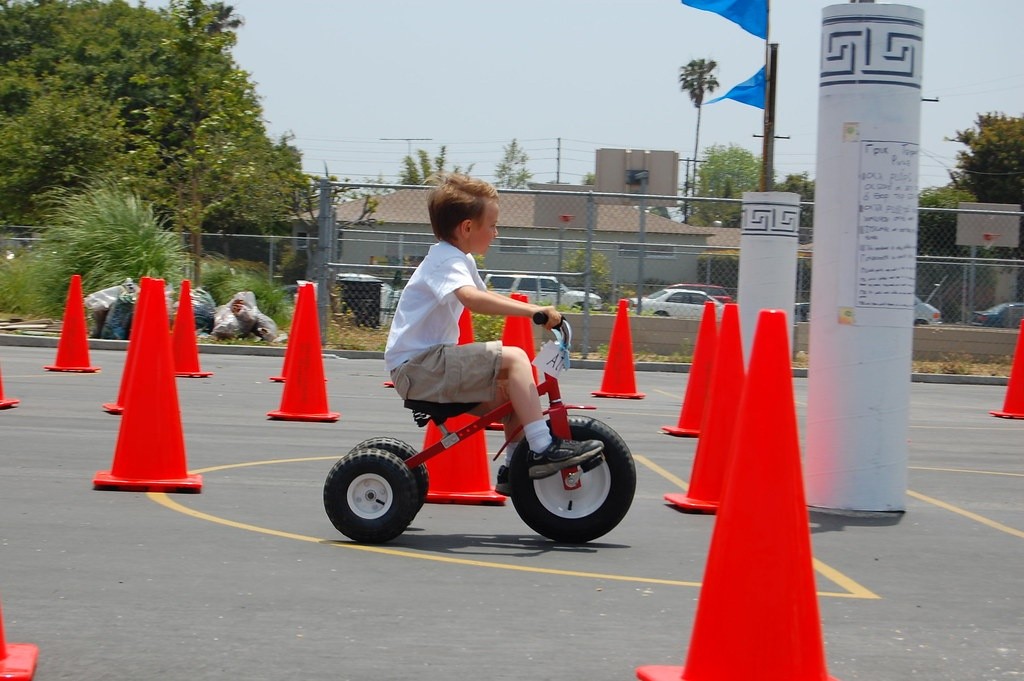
top-left (337, 274), bottom-right (384, 327)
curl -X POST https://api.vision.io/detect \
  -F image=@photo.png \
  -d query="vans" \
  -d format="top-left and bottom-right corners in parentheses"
top-left (913, 295), bottom-right (942, 326)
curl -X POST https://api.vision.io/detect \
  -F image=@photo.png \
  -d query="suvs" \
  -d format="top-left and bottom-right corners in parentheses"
top-left (483, 272), bottom-right (602, 311)
top-left (667, 283), bottom-right (732, 303)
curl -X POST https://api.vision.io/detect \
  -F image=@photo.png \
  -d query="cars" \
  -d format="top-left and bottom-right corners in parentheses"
top-left (970, 301), bottom-right (1024, 328)
top-left (278, 271), bottom-right (399, 327)
top-left (630, 289), bottom-right (720, 319)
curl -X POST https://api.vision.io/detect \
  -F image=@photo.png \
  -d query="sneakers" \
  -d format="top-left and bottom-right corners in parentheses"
top-left (495, 465), bottom-right (512, 496)
top-left (527, 437), bottom-right (604, 479)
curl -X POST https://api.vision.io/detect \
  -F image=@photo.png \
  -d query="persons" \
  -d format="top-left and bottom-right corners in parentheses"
top-left (383, 173), bottom-right (604, 496)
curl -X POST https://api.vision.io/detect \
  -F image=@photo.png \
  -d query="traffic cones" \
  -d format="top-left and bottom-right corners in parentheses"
top-left (267, 282), bottom-right (341, 422)
top-left (42, 274), bottom-right (100, 373)
top-left (486, 292), bottom-right (541, 431)
top-left (634, 311), bottom-right (837, 681)
top-left (591, 299), bottom-right (646, 399)
top-left (0, 359), bottom-right (20, 409)
top-left (988, 318), bottom-right (1024, 419)
top-left (92, 276), bottom-right (212, 491)
top-left (662, 298), bottom-right (750, 513)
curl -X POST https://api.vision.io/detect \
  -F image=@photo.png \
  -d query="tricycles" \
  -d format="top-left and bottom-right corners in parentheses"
top-left (323, 310), bottom-right (637, 545)
